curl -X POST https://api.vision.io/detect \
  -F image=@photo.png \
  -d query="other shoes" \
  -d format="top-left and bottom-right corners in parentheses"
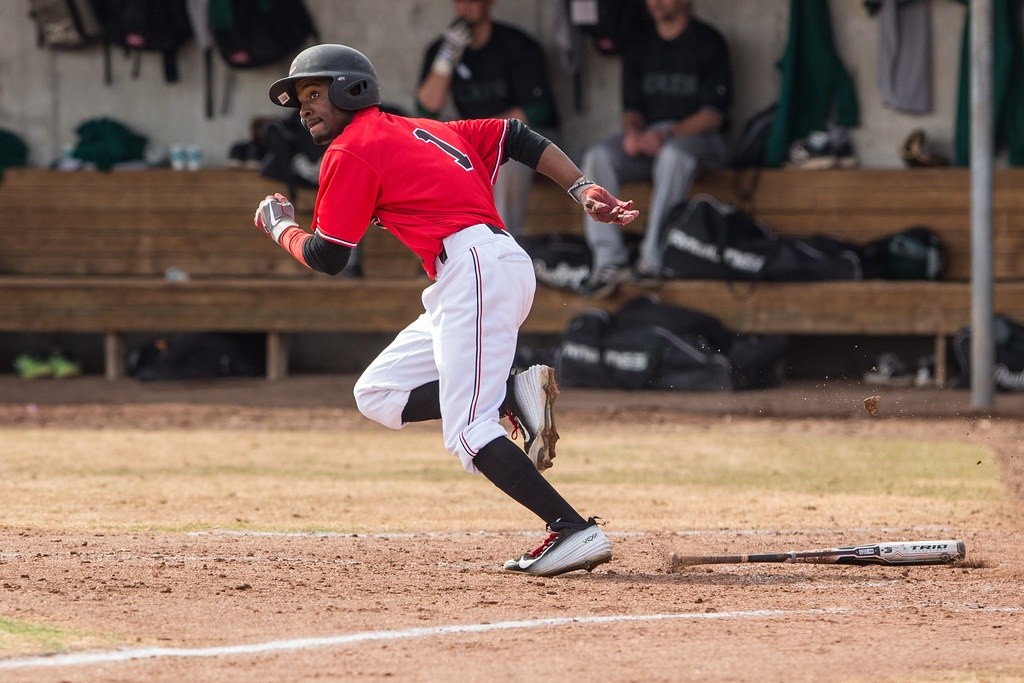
top-left (45, 356), bottom-right (79, 378)
top-left (12, 355), bottom-right (51, 379)
top-left (588, 265), bottom-right (630, 296)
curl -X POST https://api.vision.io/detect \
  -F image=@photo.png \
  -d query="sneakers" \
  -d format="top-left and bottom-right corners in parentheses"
top-left (834, 140), bottom-right (854, 170)
top-left (503, 515), bottom-right (610, 576)
top-left (786, 133), bottom-right (834, 172)
top-left (508, 361), bottom-right (558, 472)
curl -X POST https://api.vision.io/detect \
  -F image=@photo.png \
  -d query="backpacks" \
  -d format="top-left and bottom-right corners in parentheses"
top-left (26, 0), bottom-right (322, 118)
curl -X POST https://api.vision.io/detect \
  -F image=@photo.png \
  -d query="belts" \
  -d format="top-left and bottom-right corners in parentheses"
top-left (437, 222), bottom-right (508, 263)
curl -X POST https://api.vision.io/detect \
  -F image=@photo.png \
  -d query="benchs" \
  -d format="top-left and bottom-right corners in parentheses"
top-left (0, 168), bottom-right (1024, 380)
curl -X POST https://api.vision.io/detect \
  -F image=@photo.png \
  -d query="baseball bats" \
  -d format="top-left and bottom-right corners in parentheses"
top-left (670, 539), bottom-right (968, 572)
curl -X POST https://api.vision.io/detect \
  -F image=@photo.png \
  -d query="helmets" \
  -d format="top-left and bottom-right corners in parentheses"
top-left (268, 45), bottom-right (383, 110)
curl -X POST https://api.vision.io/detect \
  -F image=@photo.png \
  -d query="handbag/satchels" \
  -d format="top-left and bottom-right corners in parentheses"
top-left (660, 192), bottom-right (770, 281)
top-left (604, 293), bottom-right (783, 392)
top-left (561, 315), bottom-right (610, 392)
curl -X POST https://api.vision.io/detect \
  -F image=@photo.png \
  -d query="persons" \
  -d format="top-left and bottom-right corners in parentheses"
top-left (579, 0), bottom-right (736, 297)
top-left (414, 0), bottom-right (562, 236)
top-left (255, 44), bottom-right (639, 580)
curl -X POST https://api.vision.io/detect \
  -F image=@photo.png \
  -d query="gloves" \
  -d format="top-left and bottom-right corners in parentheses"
top-left (430, 17), bottom-right (471, 75)
top-left (567, 174), bottom-right (640, 227)
top-left (254, 193), bottom-right (300, 246)
